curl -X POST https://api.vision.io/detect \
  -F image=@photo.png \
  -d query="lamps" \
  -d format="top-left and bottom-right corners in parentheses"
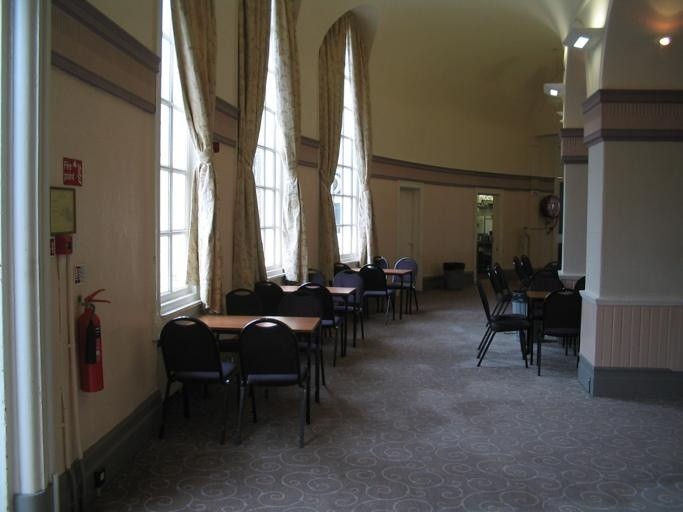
top-left (543, 83), bottom-right (566, 98)
top-left (562, 27), bottom-right (604, 51)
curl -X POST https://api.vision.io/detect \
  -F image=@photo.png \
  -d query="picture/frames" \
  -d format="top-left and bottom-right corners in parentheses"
top-left (50, 187), bottom-right (76, 236)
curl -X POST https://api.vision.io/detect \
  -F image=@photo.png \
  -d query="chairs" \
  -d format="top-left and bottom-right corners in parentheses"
top-left (476, 254), bottom-right (586, 377)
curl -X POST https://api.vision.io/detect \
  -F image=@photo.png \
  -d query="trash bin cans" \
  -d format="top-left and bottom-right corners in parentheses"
top-left (443, 262), bottom-right (465, 291)
top-left (511, 292), bottom-right (528, 315)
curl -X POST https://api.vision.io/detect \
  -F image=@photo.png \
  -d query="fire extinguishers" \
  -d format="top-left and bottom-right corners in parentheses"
top-left (77, 288), bottom-right (113, 392)
